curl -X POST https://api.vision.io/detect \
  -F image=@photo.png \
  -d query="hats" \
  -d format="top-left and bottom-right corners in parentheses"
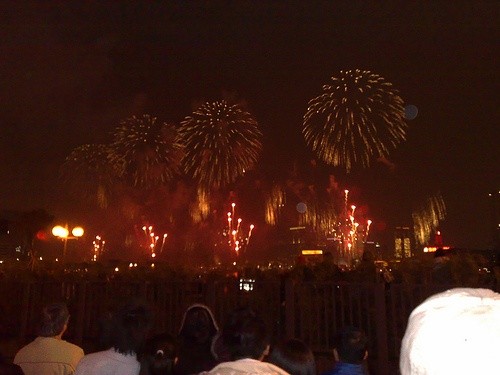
top-left (399, 287), bottom-right (500, 375)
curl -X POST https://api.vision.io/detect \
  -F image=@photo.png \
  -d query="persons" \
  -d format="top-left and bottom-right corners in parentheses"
top-left (270, 336), bottom-right (317, 375)
top-left (12, 299), bottom-right (85, 375)
top-left (331, 327), bottom-right (369, 375)
top-left (400, 286), bottom-right (500, 375)
top-left (200, 305), bottom-right (292, 375)
top-left (74, 300), bottom-right (183, 375)
top-left (0, 245), bottom-right (500, 352)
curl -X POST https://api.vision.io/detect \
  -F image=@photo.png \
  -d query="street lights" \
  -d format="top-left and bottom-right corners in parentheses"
top-left (53, 221), bottom-right (85, 266)
